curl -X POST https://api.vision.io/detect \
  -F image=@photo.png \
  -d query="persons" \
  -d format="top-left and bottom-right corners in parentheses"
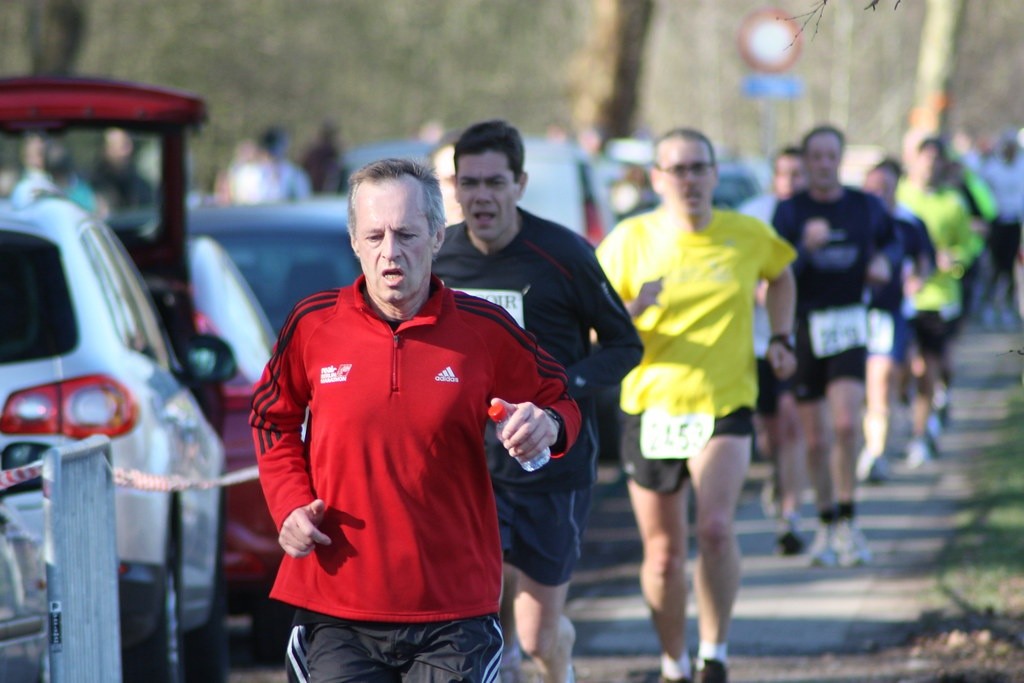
top-left (212, 123), bottom-right (347, 206)
top-left (596, 129), bottom-right (800, 683)
top-left (0, 124), bottom-right (153, 235)
top-left (432, 119), bottom-right (644, 683)
top-left (743, 126), bottom-right (1024, 572)
top-left (247, 157), bottom-right (583, 683)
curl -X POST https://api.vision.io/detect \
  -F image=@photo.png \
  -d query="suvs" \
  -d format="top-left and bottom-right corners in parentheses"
top-left (0, 167), bottom-right (238, 683)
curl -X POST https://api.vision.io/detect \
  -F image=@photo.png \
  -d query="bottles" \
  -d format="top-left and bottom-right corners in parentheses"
top-left (489, 402), bottom-right (550, 471)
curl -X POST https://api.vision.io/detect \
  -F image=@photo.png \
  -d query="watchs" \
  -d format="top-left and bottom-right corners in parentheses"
top-left (770, 332), bottom-right (796, 349)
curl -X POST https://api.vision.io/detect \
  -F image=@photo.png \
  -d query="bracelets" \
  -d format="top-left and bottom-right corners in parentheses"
top-left (545, 409), bottom-right (564, 445)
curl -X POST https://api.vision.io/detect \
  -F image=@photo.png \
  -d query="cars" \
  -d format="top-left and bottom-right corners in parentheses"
top-left (1, 73), bottom-right (295, 675)
top-left (95, 192), bottom-right (356, 340)
top-left (337, 126), bottom-right (788, 270)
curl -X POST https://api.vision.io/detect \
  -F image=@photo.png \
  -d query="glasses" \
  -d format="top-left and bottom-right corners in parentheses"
top-left (658, 162), bottom-right (714, 177)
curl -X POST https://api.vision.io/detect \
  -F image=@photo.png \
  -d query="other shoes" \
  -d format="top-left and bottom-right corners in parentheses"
top-left (828, 520), bottom-right (869, 567)
top-left (660, 648), bottom-right (692, 683)
top-left (855, 451), bottom-right (890, 481)
top-left (775, 529), bottom-right (801, 554)
top-left (695, 659), bottom-right (728, 683)
top-left (908, 435), bottom-right (935, 470)
